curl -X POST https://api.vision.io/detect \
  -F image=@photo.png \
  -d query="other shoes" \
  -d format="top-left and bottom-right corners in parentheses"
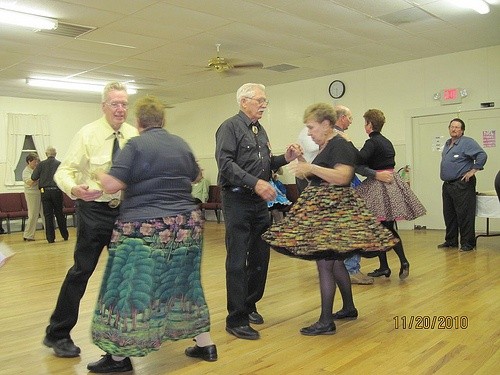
top-left (349, 272), bottom-right (375, 285)
top-left (300, 321), bottom-right (336, 336)
top-left (331, 308), bottom-right (358, 321)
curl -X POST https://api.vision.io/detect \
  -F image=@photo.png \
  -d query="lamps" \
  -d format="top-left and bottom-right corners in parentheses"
top-left (25, 78), bottom-right (136, 94)
top-left (0, 10), bottom-right (59, 30)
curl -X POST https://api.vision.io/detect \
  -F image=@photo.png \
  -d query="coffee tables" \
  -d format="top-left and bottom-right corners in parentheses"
top-left (474, 195), bottom-right (500, 248)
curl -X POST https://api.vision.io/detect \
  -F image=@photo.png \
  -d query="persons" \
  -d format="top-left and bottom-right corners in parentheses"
top-left (266, 166), bottom-right (292, 225)
top-left (21, 153), bottom-right (48, 240)
top-left (86, 96), bottom-right (218, 374)
top-left (261, 100), bottom-right (402, 338)
top-left (437, 118), bottom-right (488, 253)
top-left (359, 108), bottom-right (427, 280)
top-left (296, 124), bottom-right (320, 194)
top-left (190, 170), bottom-right (211, 205)
top-left (215, 81), bottom-right (303, 341)
top-left (42, 81), bottom-right (206, 358)
top-left (31, 145), bottom-right (69, 244)
top-left (317, 104), bottom-right (394, 285)
top-left (495, 169), bottom-right (500, 203)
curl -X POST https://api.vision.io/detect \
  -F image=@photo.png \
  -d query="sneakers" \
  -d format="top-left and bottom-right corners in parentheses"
top-left (42, 335), bottom-right (81, 357)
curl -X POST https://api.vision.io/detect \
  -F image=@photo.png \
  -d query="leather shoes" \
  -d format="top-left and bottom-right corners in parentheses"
top-left (247, 311), bottom-right (264, 325)
top-left (63, 237), bottom-right (68, 241)
top-left (87, 352), bottom-right (134, 374)
top-left (226, 323), bottom-right (260, 340)
top-left (437, 241), bottom-right (458, 249)
top-left (47, 240), bottom-right (55, 243)
top-left (185, 344), bottom-right (218, 362)
top-left (459, 246), bottom-right (473, 252)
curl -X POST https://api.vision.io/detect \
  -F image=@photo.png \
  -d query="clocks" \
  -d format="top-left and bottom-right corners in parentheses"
top-left (329, 80), bottom-right (345, 99)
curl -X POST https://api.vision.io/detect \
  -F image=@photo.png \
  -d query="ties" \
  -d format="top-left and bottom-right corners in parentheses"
top-left (112, 131), bottom-right (120, 165)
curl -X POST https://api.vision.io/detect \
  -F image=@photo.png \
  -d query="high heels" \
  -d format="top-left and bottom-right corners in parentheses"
top-left (368, 268), bottom-right (391, 278)
top-left (23, 238), bottom-right (35, 241)
top-left (399, 262), bottom-right (409, 279)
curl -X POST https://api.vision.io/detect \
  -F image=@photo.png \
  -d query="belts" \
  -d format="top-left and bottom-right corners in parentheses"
top-left (222, 186), bottom-right (259, 198)
top-left (75, 199), bottom-right (121, 209)
top-left (43, 187), bottom-right (58, 190)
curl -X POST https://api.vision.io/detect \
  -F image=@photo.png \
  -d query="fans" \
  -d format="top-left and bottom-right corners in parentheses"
top-left (186, 43), bottom-right (264, 83)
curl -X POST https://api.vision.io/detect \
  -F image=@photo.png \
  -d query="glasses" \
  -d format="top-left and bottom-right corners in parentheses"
top-left (242, 95), bottom-right (269, 104)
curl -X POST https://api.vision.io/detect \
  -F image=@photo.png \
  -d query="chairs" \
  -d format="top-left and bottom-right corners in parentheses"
top-left (0, 184), bottom-right (303, 235)
